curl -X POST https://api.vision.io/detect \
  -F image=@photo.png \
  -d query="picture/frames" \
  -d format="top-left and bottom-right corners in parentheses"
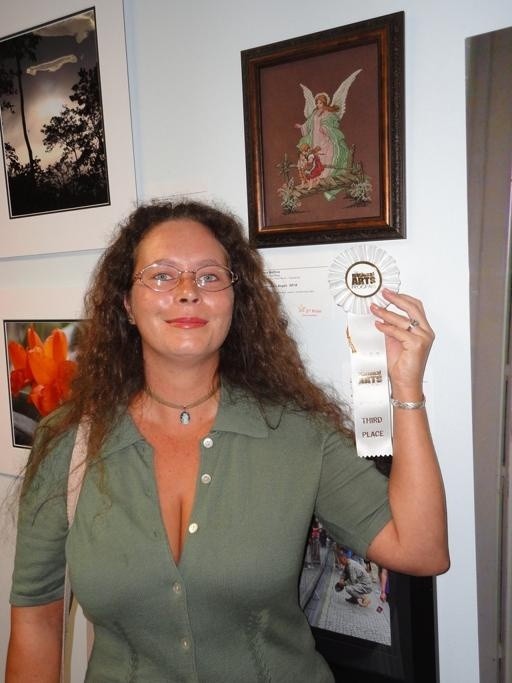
top-left (298, 509), bottom-right (438, 680)
top-left (1, 283), bottom-right (135, 478)
top-left (240, 10), bottom-right (404, 252)
top-left (0, 1), bottom-right (133, 260)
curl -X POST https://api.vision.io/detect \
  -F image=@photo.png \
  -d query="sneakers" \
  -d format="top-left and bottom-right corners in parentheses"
top-left (345, 596), bottom-right (371, 607)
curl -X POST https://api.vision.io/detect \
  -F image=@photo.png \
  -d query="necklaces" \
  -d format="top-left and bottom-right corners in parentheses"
top-left (141, 380), bottom-right (225, 427)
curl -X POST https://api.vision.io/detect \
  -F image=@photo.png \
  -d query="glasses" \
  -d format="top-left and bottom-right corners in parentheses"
top-left (131, 261), bottom-right (238, 294)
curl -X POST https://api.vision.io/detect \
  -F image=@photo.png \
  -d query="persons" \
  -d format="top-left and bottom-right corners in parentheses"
top-left (296, 152), bottom-right (309, 185)
top-left (294, 90), bottom-right (354, 184)
top-left (307, 520), bottom-right (392, 608)
top-left (299, 142), bottom-right (322, 190)
top-left (2, 194), bottom-right (451, 683)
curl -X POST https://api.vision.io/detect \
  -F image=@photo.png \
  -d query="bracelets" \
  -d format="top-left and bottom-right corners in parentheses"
top-left (391, 397), bottom-right (430, 408)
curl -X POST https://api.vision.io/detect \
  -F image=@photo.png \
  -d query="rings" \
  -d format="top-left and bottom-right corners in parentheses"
top-left (404, 319), bottom-right (420, 333)
